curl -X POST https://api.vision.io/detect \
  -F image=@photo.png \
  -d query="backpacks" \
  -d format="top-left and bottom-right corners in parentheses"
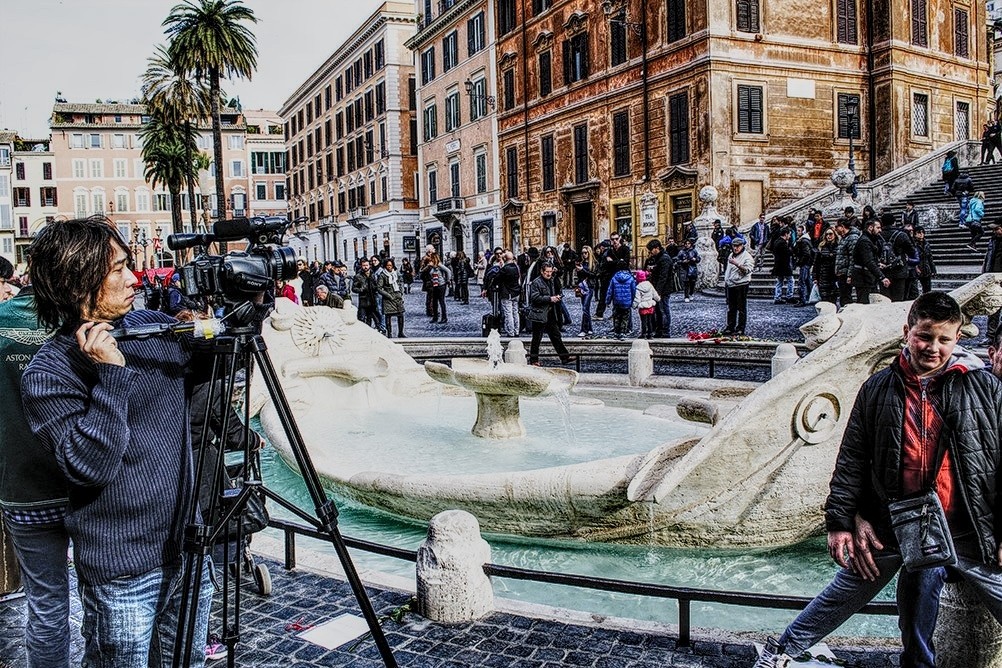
top-left (717, 243), bottom-right (732, 263)
top-left (942, 158), bottom-right (954, 173)
top-left (878, 231), bottom-right (909, 280)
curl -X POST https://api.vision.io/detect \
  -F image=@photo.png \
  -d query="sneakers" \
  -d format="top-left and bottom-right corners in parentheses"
top-left (959, 223), bottom-right (966, 229)
top-left (204, 634), bottom-right (237, 662)
top-left (967, 243), bottom-right (977, 252)
top-left (753, 636), bottom-right (793, 668)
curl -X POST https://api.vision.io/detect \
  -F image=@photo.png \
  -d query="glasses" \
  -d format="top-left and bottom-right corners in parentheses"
top-left (547, 251), bottom-right (552, 253)
top-left (611, 238), bottom-right (619, 241)
top-left (582, 252), bottom-right (587, 255)
top-left (825, 232), bottom-right (833, 236)
top-left (298, 264), bottom-right (302, 266)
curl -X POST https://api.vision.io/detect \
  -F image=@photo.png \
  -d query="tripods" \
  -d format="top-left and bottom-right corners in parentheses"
top-left (169, 332), bottom-right (400, 668)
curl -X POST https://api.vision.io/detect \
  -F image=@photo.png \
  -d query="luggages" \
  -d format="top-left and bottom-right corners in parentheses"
top-left (482, 288), bottom-right (501, 337)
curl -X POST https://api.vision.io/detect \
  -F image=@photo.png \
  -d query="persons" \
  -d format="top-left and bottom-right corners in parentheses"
top-left (22, 214), bottom-right (263, 668)
top-left (0, 120), bottom-right (1002, 668)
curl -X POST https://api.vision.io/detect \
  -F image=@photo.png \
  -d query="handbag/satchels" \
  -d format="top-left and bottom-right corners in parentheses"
top-left (889, 489), bottom-right (957, 573)
top-left (574, 280), bottom-right (589, 297)
top-left (808, 284), bottom-right (822, 303)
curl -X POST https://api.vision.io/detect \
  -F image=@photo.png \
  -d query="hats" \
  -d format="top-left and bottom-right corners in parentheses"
top-left (331, 260), bottom-right (342, 267)
top-left (636, 269), bottom-right (649, 283)
top-left (617, 259), bottom-right (628, 270)
top-left (732, 238), bottom-right (744, 245)
top-left (881, 211), bottom-right (896, 226)
top-left (688, 238), bottom-right (696, 245)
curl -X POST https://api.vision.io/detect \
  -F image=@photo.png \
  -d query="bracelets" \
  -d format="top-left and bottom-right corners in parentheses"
top-left (738, 264), bottom-right (740, 268)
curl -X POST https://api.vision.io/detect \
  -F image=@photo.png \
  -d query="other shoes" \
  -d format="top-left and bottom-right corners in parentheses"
top-left (532, 362), bottom-right (540, 367)
top-left (563, 356), bottom-right (576, 365)
top-left (722, 328), bottom-right (744, 335)
top-left (773, 296), bottom-right (807, 308)
top-left (378, 287), bottom-right (694, 340)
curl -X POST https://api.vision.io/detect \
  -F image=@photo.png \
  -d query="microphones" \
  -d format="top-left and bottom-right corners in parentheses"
top-left (213, 218), bottom-right (287, 238)
